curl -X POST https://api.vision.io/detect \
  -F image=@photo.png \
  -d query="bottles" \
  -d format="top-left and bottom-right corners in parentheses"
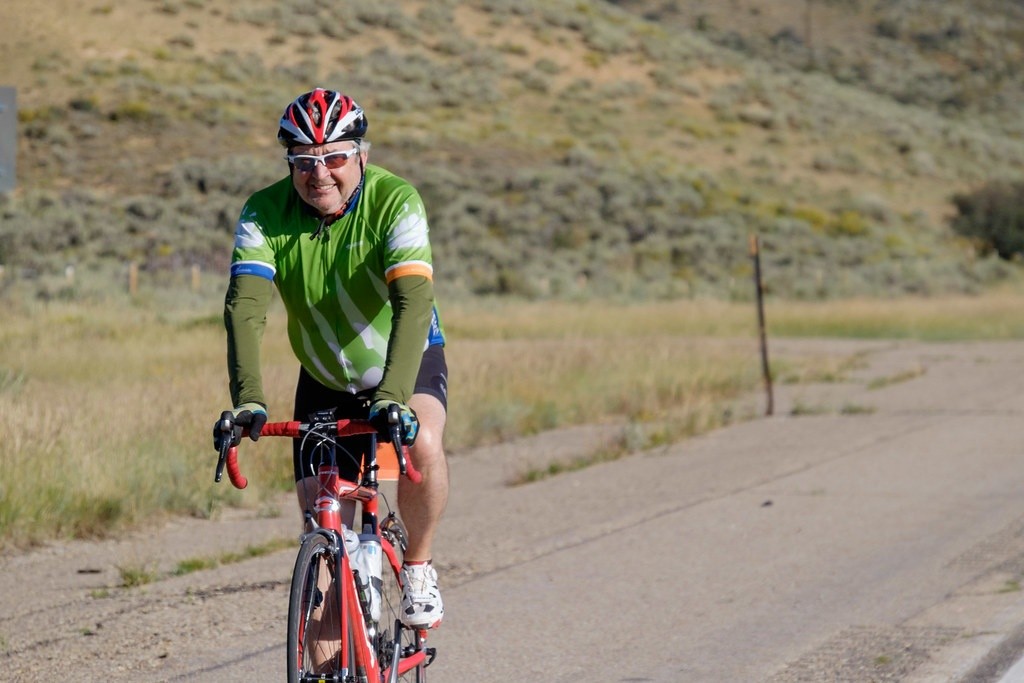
top-left (357, 523), bottom-right (382, 622)
top-left (339, 517), bottom-right (372, 609)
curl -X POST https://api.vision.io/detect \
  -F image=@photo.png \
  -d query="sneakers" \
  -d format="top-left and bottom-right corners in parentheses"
top-left (400, 562), bottom-right (442, 629)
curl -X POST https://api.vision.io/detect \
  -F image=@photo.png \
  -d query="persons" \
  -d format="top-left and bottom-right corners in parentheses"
top-left (212, 90), bottom-right (451, 683)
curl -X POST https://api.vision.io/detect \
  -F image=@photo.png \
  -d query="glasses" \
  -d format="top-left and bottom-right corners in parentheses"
top-left (284, 150), bottom-right (359, 170)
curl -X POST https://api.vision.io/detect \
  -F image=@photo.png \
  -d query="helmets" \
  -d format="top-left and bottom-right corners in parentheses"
top-left (276, 88), bottom-right (368, 146)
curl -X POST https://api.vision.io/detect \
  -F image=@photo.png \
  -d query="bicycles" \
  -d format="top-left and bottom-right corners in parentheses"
top-left (213, 408), bottom-right (436, 683)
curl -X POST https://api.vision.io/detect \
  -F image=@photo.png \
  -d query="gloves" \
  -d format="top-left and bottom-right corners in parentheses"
top-left (370, 399), bottom-right (420, 444)
top-left (213, 403), bottom-right (270, 450)
